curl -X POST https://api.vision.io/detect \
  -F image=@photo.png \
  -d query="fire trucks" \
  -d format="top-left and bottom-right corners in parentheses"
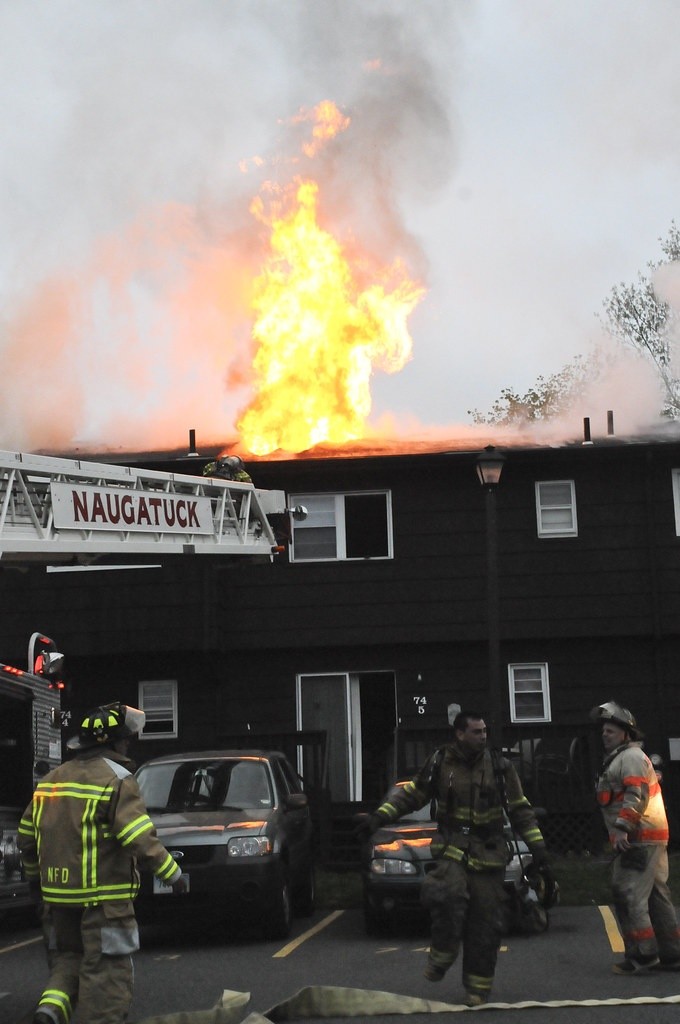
top-left (0, 450), bottom-right (308, 917)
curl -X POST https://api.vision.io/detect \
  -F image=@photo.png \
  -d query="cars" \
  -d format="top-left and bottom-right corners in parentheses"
top-left (362, 778), bottom-right (542, 936)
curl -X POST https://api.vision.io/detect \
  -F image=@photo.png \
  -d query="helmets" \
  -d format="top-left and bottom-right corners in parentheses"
top-left (65, 700), bottom-right (137, 749)
top-left (519, 861), bottom-right (559, 910)
top-left (597, 705), bottom-right (644, 742)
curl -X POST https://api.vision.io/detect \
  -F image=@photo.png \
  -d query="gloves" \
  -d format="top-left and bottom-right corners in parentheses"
top-left (29, 880), bottom-right (44, 903)
top-left (353, 815), bottom-right (382, 843)
top-left (532, 848), bottom-right (550, 866)
top-left (173, 877), bottom-right (188, 898)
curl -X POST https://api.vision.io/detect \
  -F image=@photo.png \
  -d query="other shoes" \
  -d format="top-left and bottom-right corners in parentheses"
top-left (613, 951), bottom-right (660, 975)
top-left (424, 961), bottom-right (446, 982)
top-left (464, 992), bottom-right (487, 1008)
top-left (34, 1012), bottom-right (53, 1024)
top-left (657, 955), bottom-right (680, 971)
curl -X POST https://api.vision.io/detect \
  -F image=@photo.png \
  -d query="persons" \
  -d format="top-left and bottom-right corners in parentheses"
top-left (17, 705), bottom-right (187, 1024)
top-left (596, 703), bottom-right (678, 975)
top-left (347, 712), bottom-right (547, 1007)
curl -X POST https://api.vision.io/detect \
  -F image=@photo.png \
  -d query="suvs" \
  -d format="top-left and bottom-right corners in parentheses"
top-left (130, 748), bottom-right (317, 942)
